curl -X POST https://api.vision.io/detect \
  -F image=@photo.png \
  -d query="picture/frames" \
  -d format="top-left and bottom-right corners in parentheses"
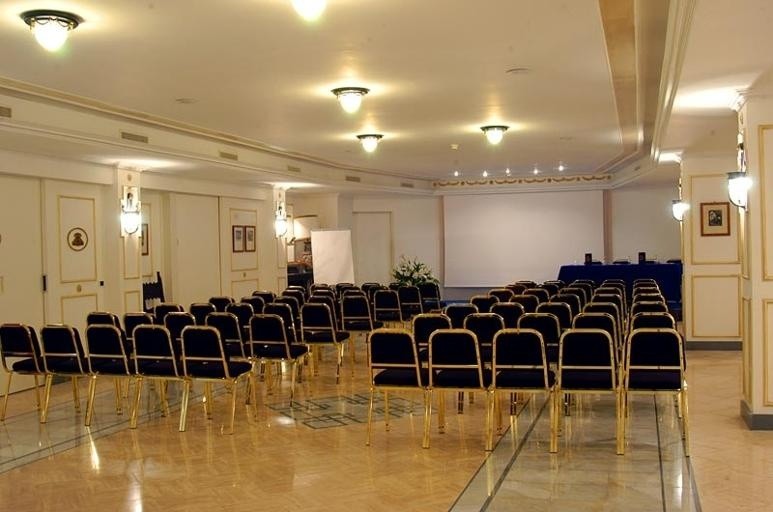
top-left (142, 224), bottom-right (148, 256)
top-left (700, 202), bottom-right (730, 236)
top-left (245, 226), bottom-right (255, 252)
top-left (232, 225), bottom-right (244, 252)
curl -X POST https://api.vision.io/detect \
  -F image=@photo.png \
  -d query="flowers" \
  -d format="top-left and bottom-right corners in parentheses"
top-left (391, 255), bottom-right (440, 286)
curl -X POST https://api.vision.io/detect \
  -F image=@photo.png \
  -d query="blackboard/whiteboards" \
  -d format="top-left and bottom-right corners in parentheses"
top-left (311, 227), bottom-right (355, 286)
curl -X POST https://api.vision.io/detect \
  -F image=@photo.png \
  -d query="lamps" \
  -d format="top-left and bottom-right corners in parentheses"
top-left (275, 201), bottom-right (288, 238)
top-left (727, 172), bottom-right (748, 211)
top-left (120, 185), bottom-right (141, 238)
top-left (480, 125), bottom-right (508, 145)
top-left (331, 87), bottom-right (369, 114)
top-left (357, 134), bottom-right (383, 151)
top-left (672, 200), bottom-right (683, 222)
top-left (20, 9), bottom-right (85, 51)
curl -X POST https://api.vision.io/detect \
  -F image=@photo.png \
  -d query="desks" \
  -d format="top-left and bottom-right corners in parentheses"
top-left (558, 264), bottom-right (682, 320)
